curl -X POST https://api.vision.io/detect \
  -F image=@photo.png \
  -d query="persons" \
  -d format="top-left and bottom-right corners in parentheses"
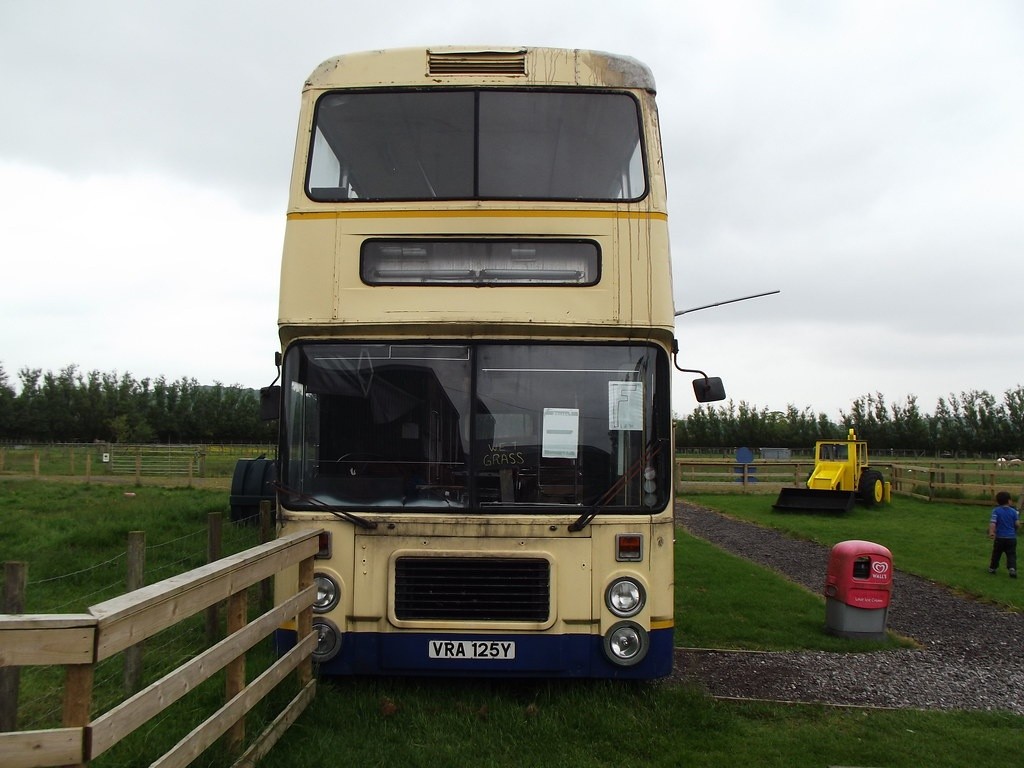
top-left (989, 491), bottom-right (1020, 578)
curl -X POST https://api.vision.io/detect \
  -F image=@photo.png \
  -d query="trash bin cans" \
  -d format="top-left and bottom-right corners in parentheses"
top-left (825, 540), bottom-right (894, 642)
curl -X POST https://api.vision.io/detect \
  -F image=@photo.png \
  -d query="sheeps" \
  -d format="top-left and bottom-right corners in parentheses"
top-left (996, 457), bottom-right (1022, 470)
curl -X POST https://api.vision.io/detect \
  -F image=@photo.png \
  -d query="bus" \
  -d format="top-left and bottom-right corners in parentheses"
top-left (226, 41), bottom-right (727, 692)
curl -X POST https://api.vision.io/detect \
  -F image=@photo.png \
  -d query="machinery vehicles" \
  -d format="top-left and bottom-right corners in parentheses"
top-left (768, 427), bottom-right (887, 516)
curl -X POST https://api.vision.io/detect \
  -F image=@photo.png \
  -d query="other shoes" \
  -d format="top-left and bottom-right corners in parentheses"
top-left (988, 567), bottom-right (996, 574)
top-left (1009, 568), bottom-right (1016, 578)
